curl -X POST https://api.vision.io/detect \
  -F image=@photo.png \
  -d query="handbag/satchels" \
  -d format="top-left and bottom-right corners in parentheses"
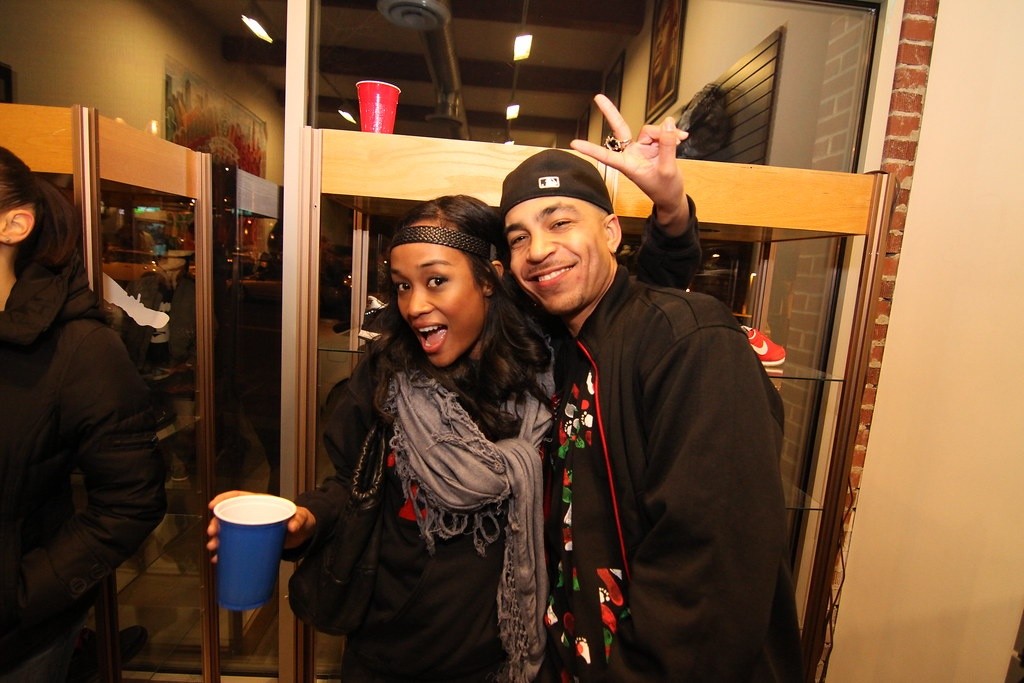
top-left (287, 420), bottom-right (389, 637)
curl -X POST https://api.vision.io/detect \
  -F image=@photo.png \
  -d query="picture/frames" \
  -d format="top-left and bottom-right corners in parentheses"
top-left (645, 0), bottom-right (687, 124)
top-left (601, 49), bottom-right (626, 147)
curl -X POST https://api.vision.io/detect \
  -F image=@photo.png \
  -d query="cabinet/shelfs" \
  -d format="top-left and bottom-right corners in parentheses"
top-left (0, 102), bottom-right (895, 683)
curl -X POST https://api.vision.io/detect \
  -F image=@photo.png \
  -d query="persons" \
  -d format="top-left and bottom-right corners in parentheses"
top-left (320, 248), bottom-right (351, 316)
top-left (500, 148), bottom-right (802, 683)
top-left (0, 146), bottom-right (169, 683)
top-left (208, 94), bottom-right (702, 683)
top-left (169, 210), bottom-right (235, 481)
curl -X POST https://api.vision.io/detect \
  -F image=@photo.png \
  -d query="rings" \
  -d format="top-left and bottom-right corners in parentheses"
top-left (604, 134), bottom-right (632, 153)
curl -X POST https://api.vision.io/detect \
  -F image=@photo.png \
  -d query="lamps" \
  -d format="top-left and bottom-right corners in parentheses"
top-left (241, 9), bottom-right (274, 43)
top-left (338, 100), bottom-right (360, 125)
top-left (426, 91), bottom-right (464, 127)
top-left (376, 0), bottom-right (451, 30)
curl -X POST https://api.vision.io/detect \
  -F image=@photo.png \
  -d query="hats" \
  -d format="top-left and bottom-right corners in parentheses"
top-left (500, 148), bottom-right (615, 227)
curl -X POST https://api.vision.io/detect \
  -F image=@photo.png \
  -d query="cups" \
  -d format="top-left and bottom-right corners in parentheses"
top-left (213, 494), bottom-right (297, 611)
top-left (355, 80), bottom-right (401, 135)
